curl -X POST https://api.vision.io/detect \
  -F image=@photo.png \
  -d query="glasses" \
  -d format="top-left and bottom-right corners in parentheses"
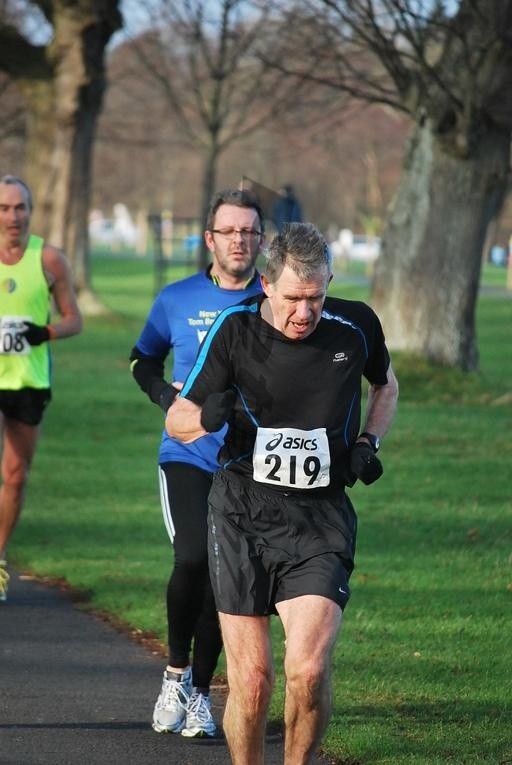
top-left (213, 226), bottom-right (262, 241)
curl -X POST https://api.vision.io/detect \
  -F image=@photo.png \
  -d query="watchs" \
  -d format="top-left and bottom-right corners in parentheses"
top-left (359, 432), bottom-right (381, 454)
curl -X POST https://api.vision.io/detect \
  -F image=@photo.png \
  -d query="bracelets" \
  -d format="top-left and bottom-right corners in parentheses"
top-left (45, 324), bottom-right (56, 340)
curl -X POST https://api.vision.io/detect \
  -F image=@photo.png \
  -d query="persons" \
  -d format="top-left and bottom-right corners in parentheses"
top-left (273, 184), bottom-right (303, 232)
top-left (0, 174), bottom-right (83, 600)
top-left (165, 221), bottom-right (399, 765)
top-left (132, 188), bottom-right (265, 738)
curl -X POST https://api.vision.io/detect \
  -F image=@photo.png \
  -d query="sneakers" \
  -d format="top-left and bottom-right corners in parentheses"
top-left (181, 686), bottom-right (217, 738)
top-left (151, 665), bottom-right (193, 735)
top-left (0, 551), bottom-right (10, 601)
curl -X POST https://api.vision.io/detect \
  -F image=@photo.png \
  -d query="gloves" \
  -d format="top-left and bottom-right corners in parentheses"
top-left (18, 321), bottom-right (51, 347)
top-left (200, 389), bottom-right (234, 433)
top-left (348, 442), bottom-right (383, 486)
